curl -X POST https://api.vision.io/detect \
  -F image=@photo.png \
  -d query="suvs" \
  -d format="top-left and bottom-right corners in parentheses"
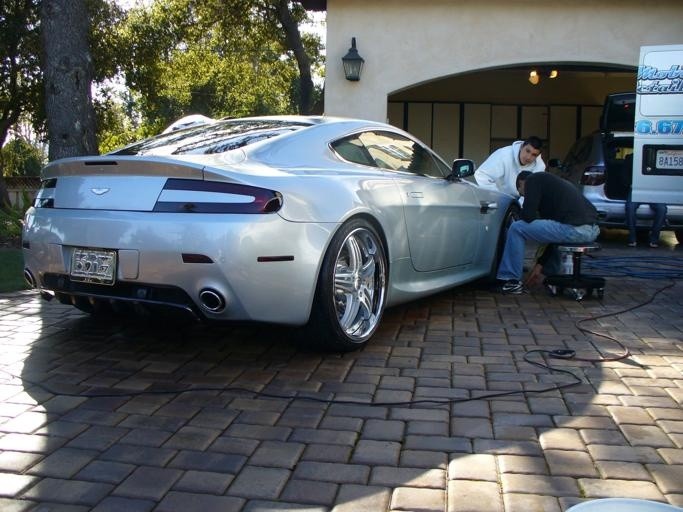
top-left (548, 92), bottom-right (683, 243)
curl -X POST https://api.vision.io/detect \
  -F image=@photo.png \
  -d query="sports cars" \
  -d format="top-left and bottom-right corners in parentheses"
top-left (21, 115), bottom-right (520, 350)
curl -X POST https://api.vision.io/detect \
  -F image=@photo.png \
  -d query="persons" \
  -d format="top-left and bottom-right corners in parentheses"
top-left (473, 136), bottom-right (547, 209)
top-left (622, 154), bottom-right (667, 248)
top-left (496, 171), bottom-right (602, 295)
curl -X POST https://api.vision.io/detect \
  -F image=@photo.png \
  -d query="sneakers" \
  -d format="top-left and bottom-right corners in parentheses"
top-left (489, 279), bottom-right (525, 295)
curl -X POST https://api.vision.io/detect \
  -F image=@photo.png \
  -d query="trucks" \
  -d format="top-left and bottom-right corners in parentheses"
top-left (632, 44), bottom-right (683, 207)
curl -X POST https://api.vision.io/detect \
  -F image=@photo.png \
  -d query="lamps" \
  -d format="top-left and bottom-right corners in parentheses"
top-left (339, 33), bottom-right (367, 81)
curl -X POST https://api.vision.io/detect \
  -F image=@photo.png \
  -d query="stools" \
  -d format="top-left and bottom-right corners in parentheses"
top-left (543, 239), bottom-right (608, 303)
